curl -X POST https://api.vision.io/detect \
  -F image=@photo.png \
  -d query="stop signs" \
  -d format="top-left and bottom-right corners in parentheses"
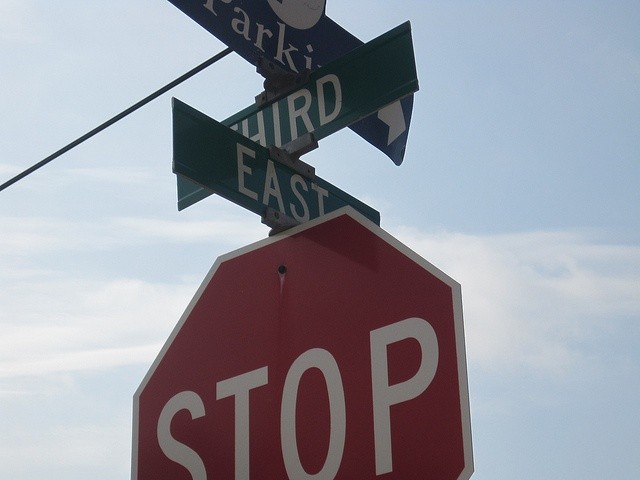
top-left (130, 205), bottom-right (473, 480)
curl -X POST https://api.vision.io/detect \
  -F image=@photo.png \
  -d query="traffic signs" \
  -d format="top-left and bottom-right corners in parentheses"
top-left (171, 97), bottom-right (381, 274)
top-left (168, 0), bottom-right (414, 166)
top-left (177, 20), bottom-right (420, 211)
